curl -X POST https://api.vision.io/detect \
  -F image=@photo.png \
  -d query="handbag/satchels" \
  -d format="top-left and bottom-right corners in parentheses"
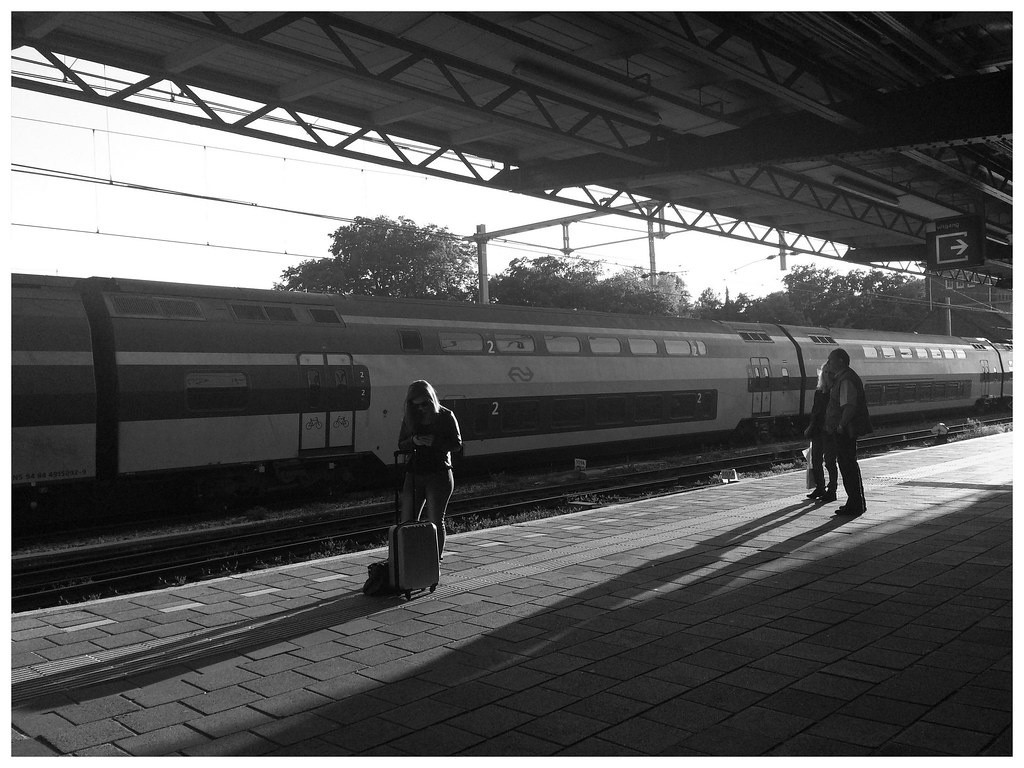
top-left (802, 438), bottom-right (816, 489)
top-left (362, 557), bottom-right (390, 595)
top-left (802, 441), bottom-right (813, 468)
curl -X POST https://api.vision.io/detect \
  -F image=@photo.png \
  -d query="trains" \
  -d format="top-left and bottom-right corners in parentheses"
top-left (11, 272), bottom-right (1014, 523)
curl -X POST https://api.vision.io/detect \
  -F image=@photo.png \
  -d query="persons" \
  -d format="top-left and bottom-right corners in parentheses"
top-left (398, 380), bottom-right (462, 560)
top-left (804, 348), bottom-right (869, 516)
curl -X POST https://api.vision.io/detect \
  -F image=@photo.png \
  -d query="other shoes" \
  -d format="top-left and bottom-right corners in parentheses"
top-left (817, 490), bottom-right (837, 502)
top-left (806, 486), bottom-right (826, 498)
top-left (839, 496), bottom-right (867, 512)
top-left (835, 496), bottom-right (864, 515)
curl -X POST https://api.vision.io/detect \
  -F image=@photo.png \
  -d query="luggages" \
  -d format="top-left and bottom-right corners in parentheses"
top-left (389, 449), bottom-right (440, 601)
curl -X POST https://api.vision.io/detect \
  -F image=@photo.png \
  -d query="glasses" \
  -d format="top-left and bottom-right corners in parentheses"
top-left (412, 401), bottom-right (429, 408)
